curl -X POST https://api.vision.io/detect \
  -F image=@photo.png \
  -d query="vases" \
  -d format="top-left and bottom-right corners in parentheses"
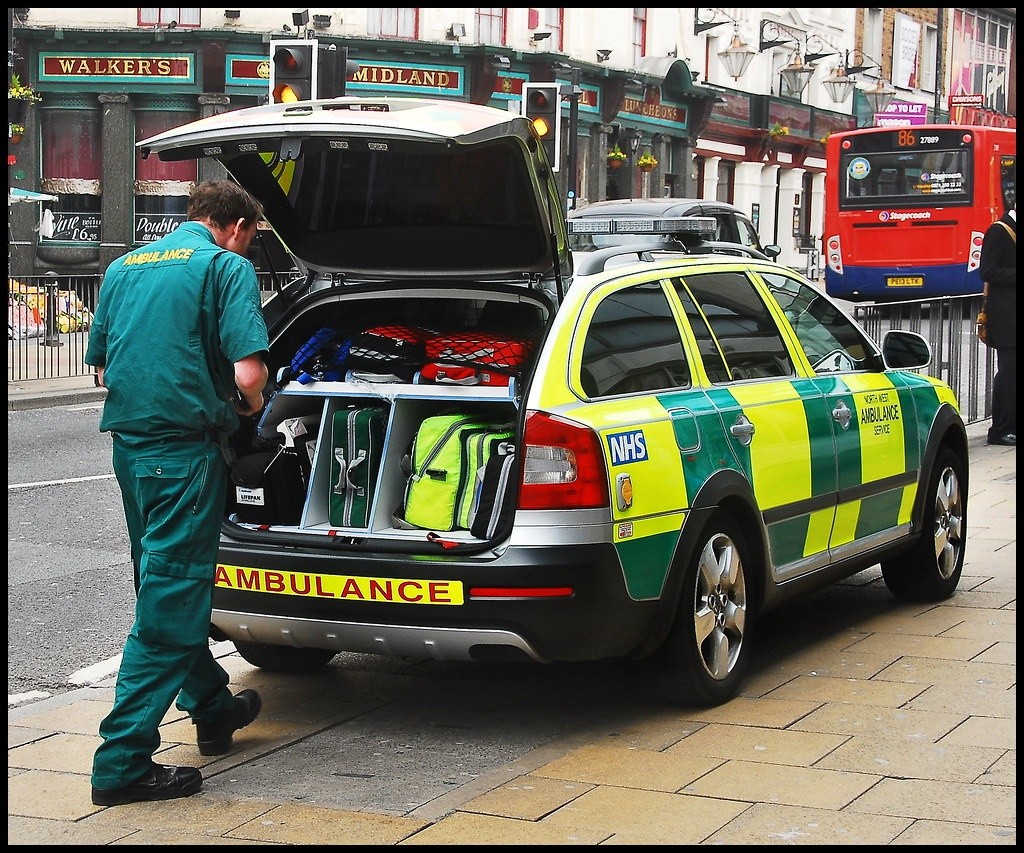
top-left (10, 132), bottom-right (25, 145)
top-left (8, 98), bottom-right (31, 122)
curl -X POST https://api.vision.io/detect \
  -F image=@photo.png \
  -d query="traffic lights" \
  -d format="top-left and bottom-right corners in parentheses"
top-left (521, 83), bottom-right (561, 175)
top-left (267, 38), bottom-right (318, 110)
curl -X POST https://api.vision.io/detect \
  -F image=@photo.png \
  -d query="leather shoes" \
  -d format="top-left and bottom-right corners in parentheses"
top-left (987, 434), bottom-right (1016, 446)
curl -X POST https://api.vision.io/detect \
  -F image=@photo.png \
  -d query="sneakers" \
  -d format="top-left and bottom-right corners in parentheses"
top-left (91, 760), bottom-right (202, 805)
top-left (192, 689), bottom-right (261, 756)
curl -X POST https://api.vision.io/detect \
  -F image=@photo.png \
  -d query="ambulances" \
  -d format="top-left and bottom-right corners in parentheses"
top-left (132, 98), bottom-right (971, 707)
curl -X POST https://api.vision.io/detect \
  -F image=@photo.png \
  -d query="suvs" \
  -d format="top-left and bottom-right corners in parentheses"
top-left (564, 193), bottom-right (781, 275)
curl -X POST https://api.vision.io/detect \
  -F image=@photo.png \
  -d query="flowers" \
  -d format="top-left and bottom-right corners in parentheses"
top-left (9, 122), bottom-right (25, 133)
top-left (8, 72), bottom-right (43, 106)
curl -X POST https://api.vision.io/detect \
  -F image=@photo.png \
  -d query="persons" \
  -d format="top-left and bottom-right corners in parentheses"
top-left (978, 200), bottom-right (1017, 446)
top-left (84, 177), bottom-right (270, 806)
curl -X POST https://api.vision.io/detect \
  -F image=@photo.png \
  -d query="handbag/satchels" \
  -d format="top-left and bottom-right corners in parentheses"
top-left (976, 307), bottom-right (996, 349)
top-left (420, 333), bottom-right (534, 386)
top-left (345, 369), bottom-right (407, 383)
top-left (342, 326), bottom-right (434, 375)
top-left (390, 412), bottom-right (486, 532)
top-left (467, 440), bottom-right (516, 542)
top-left (287, 326), bottom-right (350, 385)
top-left (326, 403), bottom-right (386, 528)
top-left (458, 420), bottom-right (515, 529)
top-left (235, 410), bottom-right (321, 524)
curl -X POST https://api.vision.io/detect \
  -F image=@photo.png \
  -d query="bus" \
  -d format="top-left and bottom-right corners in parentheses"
top-left (820, 122), bottom-right (1018, 312)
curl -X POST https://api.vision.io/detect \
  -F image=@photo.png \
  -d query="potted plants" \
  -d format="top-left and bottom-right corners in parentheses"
top-left (635, 148), bottom-right (659, 173)
top-left (769, 121), bottom-right (789, 141)
top-left (606, 144), bottom-right (629, 168)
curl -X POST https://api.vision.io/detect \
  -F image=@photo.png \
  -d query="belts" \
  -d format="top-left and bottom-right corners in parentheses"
top-left (180, 430), bottom-right (218, 442)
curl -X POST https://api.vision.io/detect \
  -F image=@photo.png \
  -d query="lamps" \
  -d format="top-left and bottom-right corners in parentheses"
top-left (629, 126), bottom-right (642, 156)
top-left (760, 18), bottom-right (815, 95)
top-left (845, 48), bottom-right (896, 114)
top-left (804, 35), bottom-right (859, 103)
top-left (529, 32), bottom-right (552, 47)
top-left (223, 9), bottom-right (243, 28)
top-left (693, 8), bottom-right (756, 83)
top-left (445, 23), bottom-right (467, 42)
top-left (596, 49), bottom-right (612, 63)
